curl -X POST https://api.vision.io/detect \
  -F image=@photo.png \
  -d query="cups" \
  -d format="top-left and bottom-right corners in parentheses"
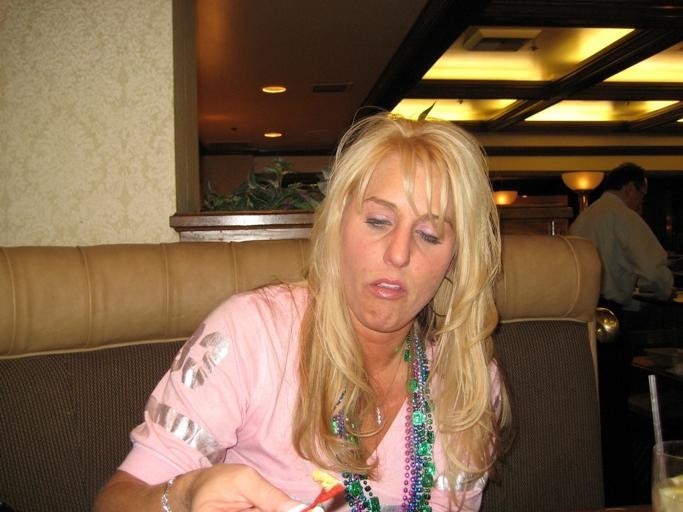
top-left (650, 439), bottom-right (683, 512)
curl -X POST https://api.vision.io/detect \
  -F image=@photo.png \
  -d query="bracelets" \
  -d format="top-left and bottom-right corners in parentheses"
top-left (157, 472), bottom-right (188, 512)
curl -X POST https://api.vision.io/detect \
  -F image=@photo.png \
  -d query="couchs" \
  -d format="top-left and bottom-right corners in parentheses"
top-left (0, 233), bottom-right (608, 511)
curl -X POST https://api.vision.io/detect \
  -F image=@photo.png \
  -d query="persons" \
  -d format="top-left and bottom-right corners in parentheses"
top-left (565, 162), bottom-right (676, 511)
top-left (92, 107), bottom-right (517, 512)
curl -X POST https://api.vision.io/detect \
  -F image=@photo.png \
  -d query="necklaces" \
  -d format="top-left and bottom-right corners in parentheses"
top-left (329, 325), bottom-right (436, 512)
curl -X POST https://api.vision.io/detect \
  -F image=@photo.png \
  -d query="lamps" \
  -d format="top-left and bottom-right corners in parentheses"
top-left (490, 190), bottom-right (518, 205)
top-left (562, 172), bottom-right (604, 194)
